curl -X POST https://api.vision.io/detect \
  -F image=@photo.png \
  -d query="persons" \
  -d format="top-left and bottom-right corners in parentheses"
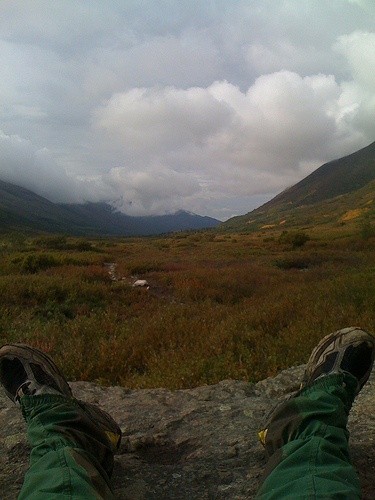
top-left (1, 326), bottom-right (374, 500)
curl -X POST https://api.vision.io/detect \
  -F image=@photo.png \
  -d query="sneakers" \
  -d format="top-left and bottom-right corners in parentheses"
top-left (1, 344), bottom-right (121, 452)
top-left (258, 326), bottom-right (375, 456)
